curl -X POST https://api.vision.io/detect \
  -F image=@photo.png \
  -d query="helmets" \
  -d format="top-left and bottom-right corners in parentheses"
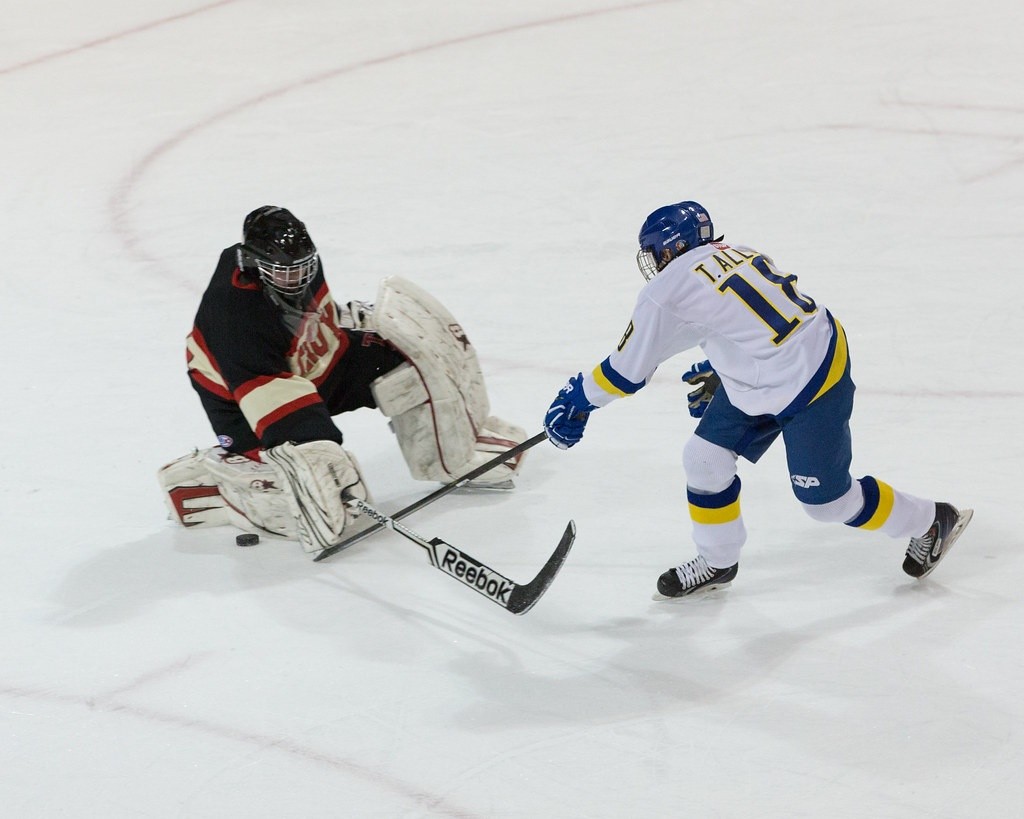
top-left (244, 205), bottom-right (318, 301)
top-left (637, 200), bottom-right (714, 282)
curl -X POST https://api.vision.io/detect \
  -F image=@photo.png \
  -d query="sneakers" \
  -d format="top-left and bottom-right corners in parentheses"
top-left (650, 556), bottom-right (738, 605)
top-left (903, 501), bottom-right (973, 587)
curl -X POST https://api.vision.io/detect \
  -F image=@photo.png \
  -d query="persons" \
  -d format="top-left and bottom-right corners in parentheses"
top-left (155, 204), bottom-right (529, 554)
top-left (543, 199), bottom-right (972, 606)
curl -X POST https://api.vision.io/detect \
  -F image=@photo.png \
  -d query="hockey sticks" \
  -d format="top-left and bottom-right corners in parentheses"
top-left (310, 410), bottom-right (588, 567)
top-left (343, 490), bottom-right (581, 618)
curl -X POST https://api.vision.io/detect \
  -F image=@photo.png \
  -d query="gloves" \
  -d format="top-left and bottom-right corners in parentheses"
top-left (682, 359), bottom-right (721, 417)
top-left (542, 372), bottom-right (599, 450)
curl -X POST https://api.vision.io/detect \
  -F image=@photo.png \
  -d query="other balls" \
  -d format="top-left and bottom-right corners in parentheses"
top-left (235, 533), bottom-right (260, 547)
